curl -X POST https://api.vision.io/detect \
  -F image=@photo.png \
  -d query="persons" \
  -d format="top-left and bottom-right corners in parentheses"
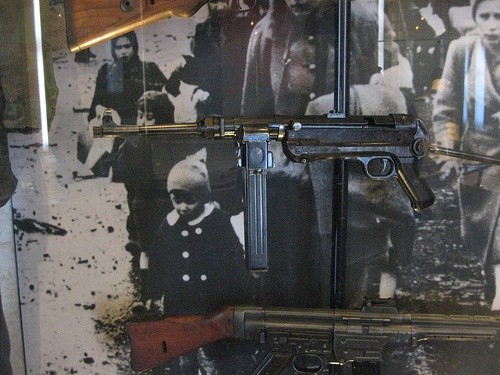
top-left (117, 89), bottom-right (182, 276)
top-left (431, 1), bottom-right (500, 311)
top-left (85, 30), bottom-right (169, 154)
top-left (240, 1), bottom-right (415, 310)
top-left (165, 1), bottom-right (260, 122)
top-left (143, 157), bottom-right (247, 321)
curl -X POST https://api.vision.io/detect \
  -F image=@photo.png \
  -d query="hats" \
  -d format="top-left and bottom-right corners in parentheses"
top-left (167, 161), bottom-right (210, 202)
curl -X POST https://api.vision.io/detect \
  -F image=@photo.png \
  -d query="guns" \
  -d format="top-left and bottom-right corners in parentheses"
top-left (92, 109), bottom-right (500, 272)
top-left (122, 304), bottom-right (500, 373)
